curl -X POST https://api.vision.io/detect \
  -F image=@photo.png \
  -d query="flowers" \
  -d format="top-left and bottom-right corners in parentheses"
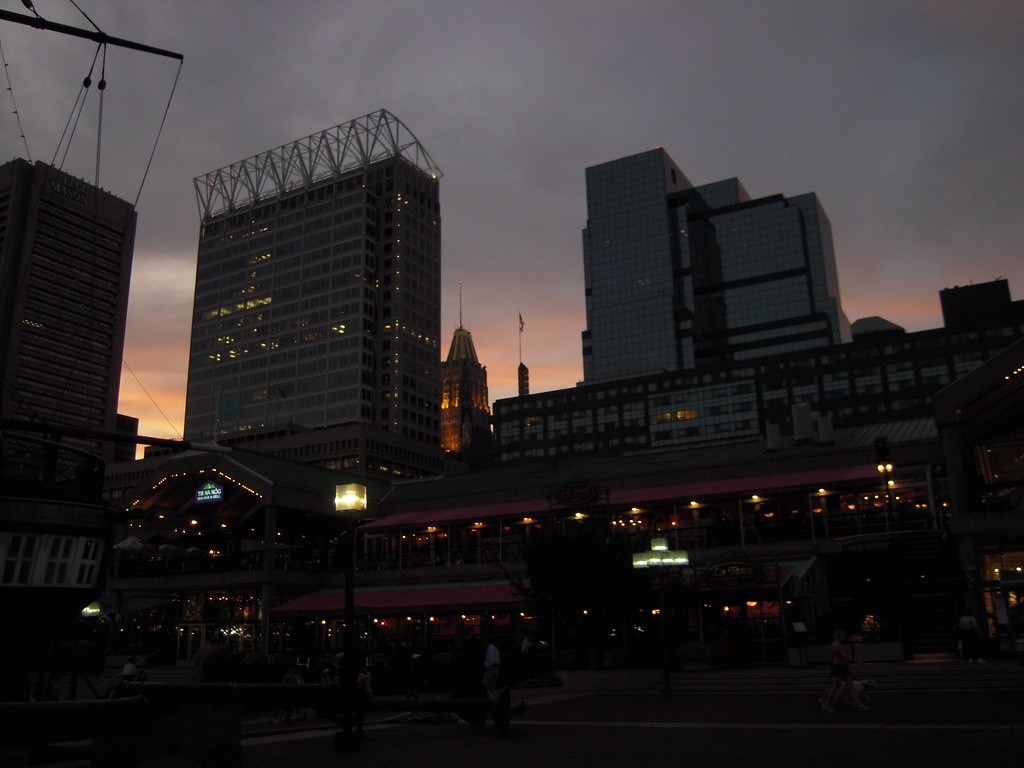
top-left (848, 614), bottom-right (889, 644)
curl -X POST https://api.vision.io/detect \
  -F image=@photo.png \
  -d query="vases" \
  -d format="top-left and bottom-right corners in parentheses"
top-left (851, 643), bottom-right (904, 663)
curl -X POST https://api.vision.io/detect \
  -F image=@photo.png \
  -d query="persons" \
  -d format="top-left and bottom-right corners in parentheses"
top-left (122, 636), bottom-right (421, 726)
top-left (958, 608), bottom-right (987, 663)
top-left (818, 630), bottom-right (867, 714)
top-left (483, 636), bottom-right (500, 697)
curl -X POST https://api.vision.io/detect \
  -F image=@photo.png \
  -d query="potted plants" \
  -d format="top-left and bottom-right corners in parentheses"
top-left (676, 642), bottom-right (718, 670)
